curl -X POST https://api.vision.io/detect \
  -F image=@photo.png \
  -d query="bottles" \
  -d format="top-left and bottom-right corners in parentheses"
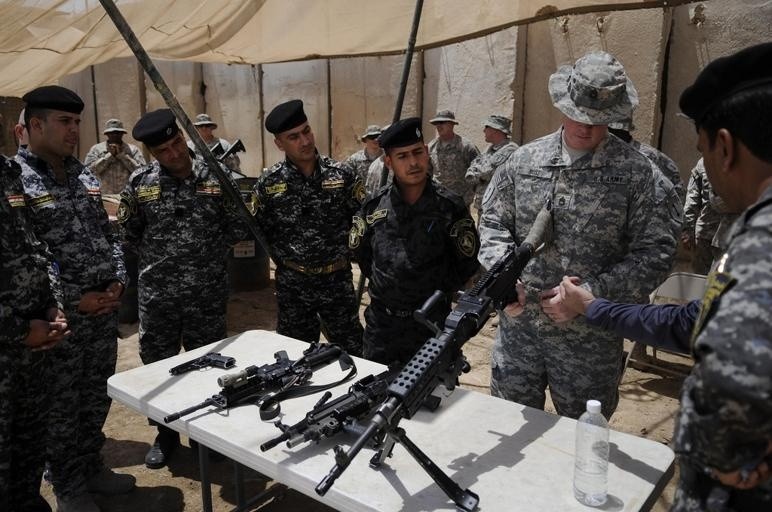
top-left (572, 398), bottom-right (611, 509)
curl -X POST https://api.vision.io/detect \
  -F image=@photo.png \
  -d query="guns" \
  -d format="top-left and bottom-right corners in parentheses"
top-left (261, 358), bottom-right (442, 455)
top-left (314, 198), bottom-right (554, 510)
top-left (209, 136), bottom-right (247, 163)
top-left (164, 340), bottom-right (347, 423)
top-left (168, 349), bottom-right (237, 375)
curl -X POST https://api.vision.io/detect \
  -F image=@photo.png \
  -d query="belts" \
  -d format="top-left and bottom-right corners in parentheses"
top-left (283, 261), bottom-right (348, 275)
top-left (370, 299), bottom-right (452, 321)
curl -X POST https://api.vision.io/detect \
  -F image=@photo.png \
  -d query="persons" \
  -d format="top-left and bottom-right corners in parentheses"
top-left (349, 117), bottom-right (478, 385)
top-left (252, 101), bottom-right (366, 360)
top-left (14, 109), bottom-right (724, 277)
top-left (668, 38), bottom-right (771, 512)
top-left (474, 49), bottom-right (684, 427)
top-left (561, 277), bottom-right (704, 355)
top-left (0, 152), bottom-right (72, 511)
top-left (23, 85), bottom-right (137, 512)
top-left (115, 109), bottom-right (247, 475)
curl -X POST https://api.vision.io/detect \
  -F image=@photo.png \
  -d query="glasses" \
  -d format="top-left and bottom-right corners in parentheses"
top-left (367, 134), bottom-right (381, 140)
top-left (432, 121), bottom-right (450, 125)
top-left (197, 125), bottom-right (211, 128)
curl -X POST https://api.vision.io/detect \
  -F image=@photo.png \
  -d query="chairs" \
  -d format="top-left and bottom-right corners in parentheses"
top-left (620, 269), bottom-right (708, 382)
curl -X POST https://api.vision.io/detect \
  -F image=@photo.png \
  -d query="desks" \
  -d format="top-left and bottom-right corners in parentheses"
top-left (107, 328), bottom-right (675, 512)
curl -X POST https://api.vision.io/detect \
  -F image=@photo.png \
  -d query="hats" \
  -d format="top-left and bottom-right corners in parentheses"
top-left (192, 114), bottom-right (218, 129)
top-left (485, 115), bottom-right (512, 135)
top-left (132, 108), bottom-right (179, 147)
top-left (22, 85), bottom-right (84, 114)
top-left (549, 43), bottom-right (771, 132)
top-left (265, 100), bottom-right (307, 135)
top-left (429, 110), bottom-right (459, 125)
top-left (361, 125), bottom-right (383, 138)
top-left (378, 117), bottom-right (423, 148)
top-left (104, 119), bottom-right (128, 135)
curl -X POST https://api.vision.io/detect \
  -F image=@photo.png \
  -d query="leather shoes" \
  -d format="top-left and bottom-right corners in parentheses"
top-left (57, 432), bottom-right (227, 511)
